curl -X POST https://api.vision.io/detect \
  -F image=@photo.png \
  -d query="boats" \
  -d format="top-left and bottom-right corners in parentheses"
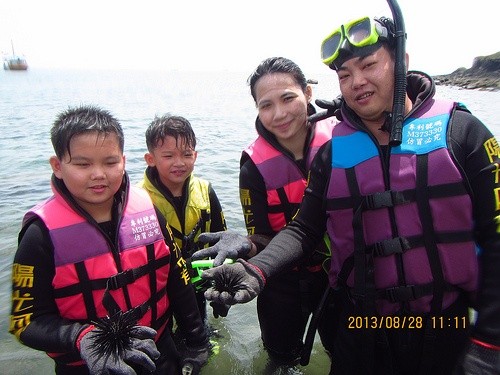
top-left (7, 58), bottom-right (28, 70)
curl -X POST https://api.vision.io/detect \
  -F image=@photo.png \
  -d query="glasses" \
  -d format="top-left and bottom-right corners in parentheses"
top-left (320, 16), bottom-right (383, 67)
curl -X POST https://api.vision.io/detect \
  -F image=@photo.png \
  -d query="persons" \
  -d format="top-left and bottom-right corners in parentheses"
top-left (201, 15), bottom-right (500, 375)
top-left (192, 57), bottom-right (341, 375)
top-left (7, 105), bottom-right (213, 375)
top-left (135, 114), bottom-right (227, 359)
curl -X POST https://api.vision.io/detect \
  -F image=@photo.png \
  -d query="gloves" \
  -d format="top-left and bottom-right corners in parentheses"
top-left (192, 230), bottom-right (255, 268)
top-left (79, 323), bottom-right (160, 375)
top-left (463, 353), bottom-right (500, 375)
top-left (202, 257), bottom-right (265, 305)
